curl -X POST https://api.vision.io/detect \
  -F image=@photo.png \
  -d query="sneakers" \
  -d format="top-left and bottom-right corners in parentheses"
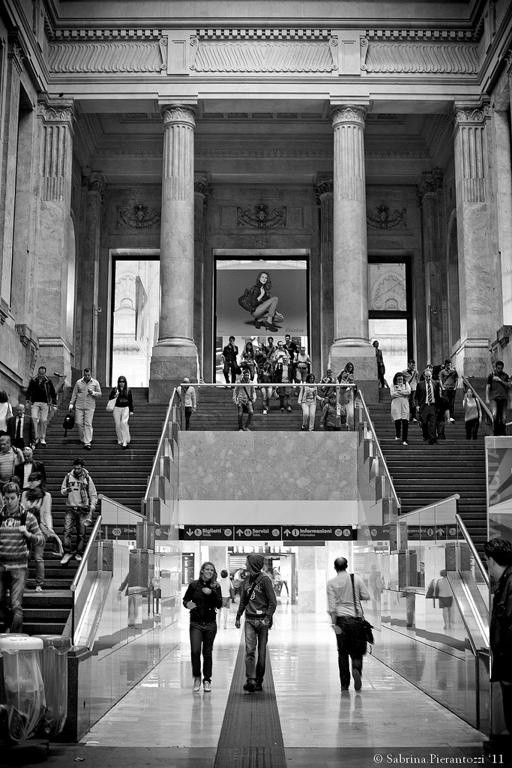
top-left (60, 553), bottom-right (82, 564)
top-left (194, 678), bottom-right (211, 692)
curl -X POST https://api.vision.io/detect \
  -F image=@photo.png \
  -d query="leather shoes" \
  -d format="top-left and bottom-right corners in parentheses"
top-left (244, 679), bottom-right (261, 692)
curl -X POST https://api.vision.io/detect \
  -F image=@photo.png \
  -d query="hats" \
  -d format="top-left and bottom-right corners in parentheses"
top-left (248, 555), bottom-right (264, 572)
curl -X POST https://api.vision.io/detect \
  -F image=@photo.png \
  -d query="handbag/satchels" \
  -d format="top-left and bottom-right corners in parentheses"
top-left (106, 398), bottom-right (117, 412)
top-left (63, 415), bottom-right (74, 429)
top-left (362, 621), bottom-right (373, 644)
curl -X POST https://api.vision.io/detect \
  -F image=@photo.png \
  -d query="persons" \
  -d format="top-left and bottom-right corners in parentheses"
top-left (233, 563), bottom-right (292, 607)
top-left (60, 458), bottom-right (99, 565)
top-left (109, 376), bottom-right (135, 450)
top-left (402, 562), bottom-right (456, 631)
top-left (68, 367), bottom-right (103, 450)
top-left (234, 552), bottom-right (278, 691)
top-left (327, 557), bottom-right (374, 690)
top-left (25, 366), bottom-right (57, 447)
top-left (482, 536), bottom-right (512, 736)
top-left (150, 568), bottom-right (178, 616)
top-left (182, 562), bottom-right (223, 692)
top-left (118, 569), bottom-right (143, 628)
top-left (221, 334), bottom-right (358, 431)
top-left (462, 387), bottom-right (483, 440)
top-left (176, 378), bottom-right (197, 431)
top-left (373, 340), bottom-right (386, 389)
top-left (217, 569), bottom-right (233, 630)
top-left (485, 361), bottom-right (511, 436)
top-left (0, 391), bottom-right (64, 636)
top-left (248, 271), bottom-right (283, 332)
top-left (390, 359), bottom-right (459, 446)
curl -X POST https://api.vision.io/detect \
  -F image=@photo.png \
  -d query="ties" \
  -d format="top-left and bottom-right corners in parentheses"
top-left (428, 382), bottom-right (432, 402)
top-left (16, 420), bottom-right (21, 441)
